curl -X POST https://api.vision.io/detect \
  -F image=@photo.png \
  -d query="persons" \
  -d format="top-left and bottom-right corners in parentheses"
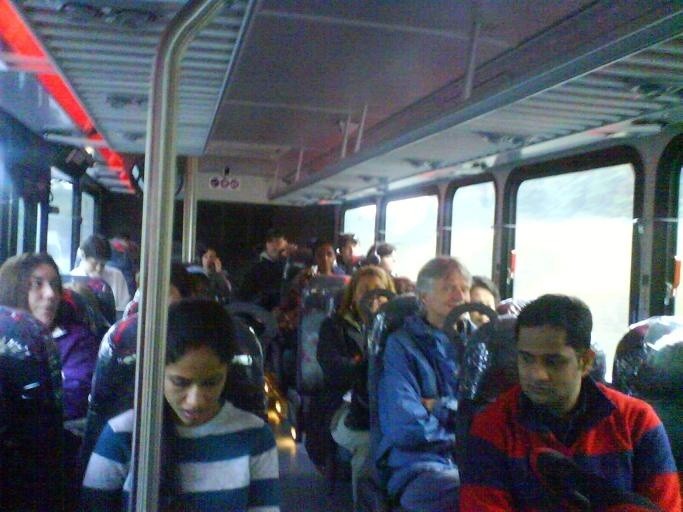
top-left (0, 218), bottom-right (682, 512)
